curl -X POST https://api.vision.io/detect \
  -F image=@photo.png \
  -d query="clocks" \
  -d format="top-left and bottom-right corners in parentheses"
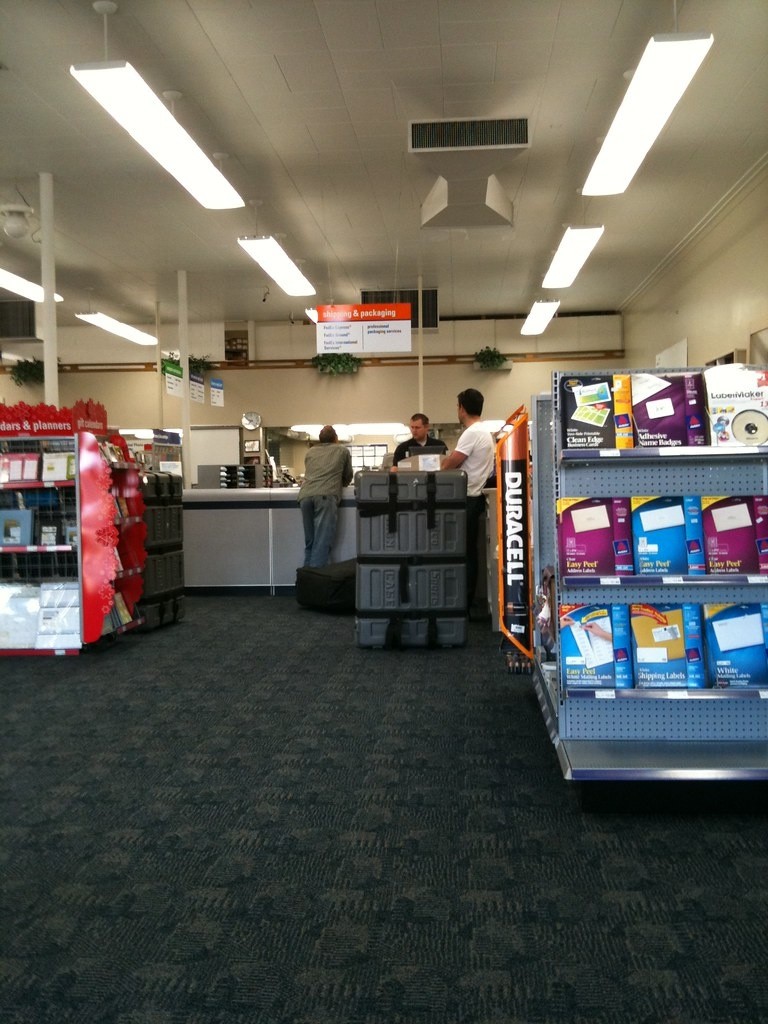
top-left (242, 412), bottom-right (262, 431)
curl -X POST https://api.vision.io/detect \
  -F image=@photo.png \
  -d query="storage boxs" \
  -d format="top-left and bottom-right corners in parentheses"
top-left (356, 559), bottom-right (467, 612)
top-left (354, 612), bottom-right (467, 645)
top-left (135, 471), bottom-right (184, 505)
top-left (132, 551), bottom-right (188, 598)
top-left (140, 505), bottom-right (185, 552)
top-left (356, 508), bottom-right (466, 560)
top-left (356, 470), bottom-right (468, 507)
top-left (130, 590), bottom-right (185, 633)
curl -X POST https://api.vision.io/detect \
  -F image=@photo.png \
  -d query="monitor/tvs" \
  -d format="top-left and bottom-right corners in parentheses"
top-left (408, 446), bottom-right (445, 457)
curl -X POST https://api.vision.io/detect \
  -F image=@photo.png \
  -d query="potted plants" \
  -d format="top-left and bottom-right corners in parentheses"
top-left (313, 355), bottom-right (361, 376)
top-left (473, 347), bottom-right (514, 372)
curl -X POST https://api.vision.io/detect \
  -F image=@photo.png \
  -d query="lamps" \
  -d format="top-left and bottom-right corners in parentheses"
top-left (69, 3), bottom-right (246, 210)
top-left (542, 190), bottom-right (607, 288)
top-left (305, 304), bottom-right (319, 325)
top-left (237, 200), bottom-right (316, 297)
top-left (74, 284), bottom-right (159, 346)
top-left (0, 268), bottom-right (64, 302)
top-left (581, 0), bottom-right (716, 195)
top-left (519, 287), bottom-right (561, 335)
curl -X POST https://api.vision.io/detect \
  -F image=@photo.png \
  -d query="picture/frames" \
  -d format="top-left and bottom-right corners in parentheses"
top-left (245, 440), bottom-right (260, 453)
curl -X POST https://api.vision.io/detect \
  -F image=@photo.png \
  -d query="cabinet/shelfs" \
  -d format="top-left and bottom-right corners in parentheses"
top-left (0, 401), bottom-right (147, 656)
top-left (530, 365), bottom-right (768, 781)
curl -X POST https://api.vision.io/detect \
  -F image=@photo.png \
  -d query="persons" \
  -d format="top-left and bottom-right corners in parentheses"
top-left (442, 387), bottom-right (495, 620)
top-left (296, 426), bottom-right (352, 569)
top-left (559, 615), bottom-right (613, 640)
top-left (389, 413), bottom-right (448, 471)
